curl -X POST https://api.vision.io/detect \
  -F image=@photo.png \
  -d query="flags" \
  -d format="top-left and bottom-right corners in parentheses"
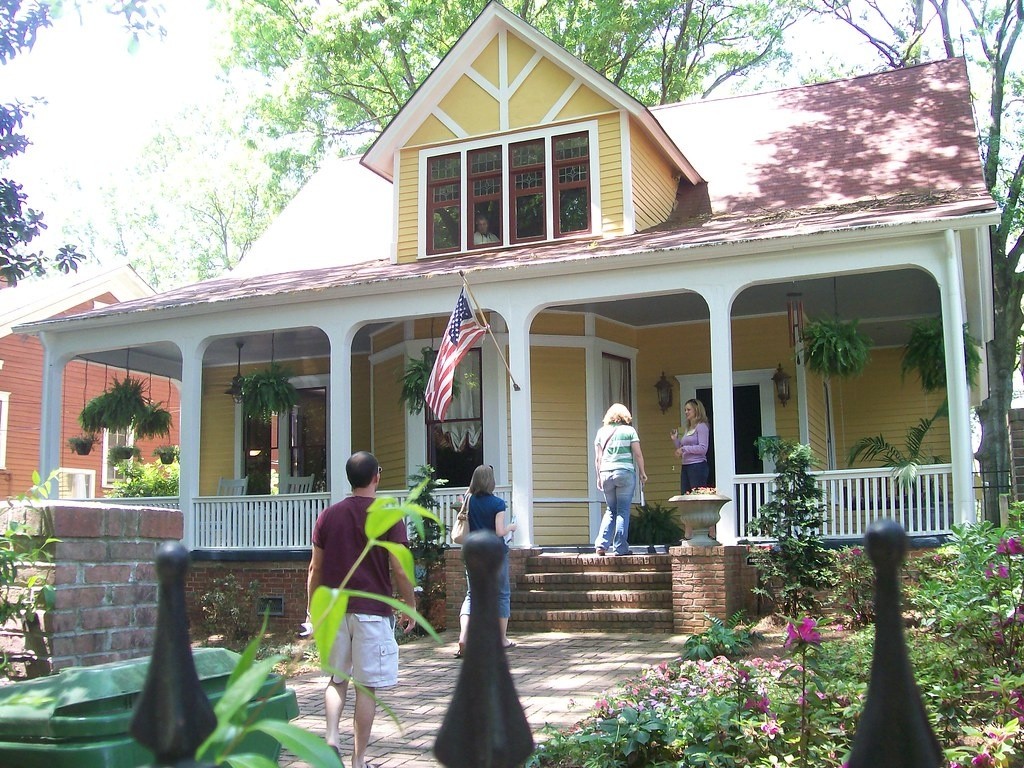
top-left (424, 281), bottom-right (486, 422)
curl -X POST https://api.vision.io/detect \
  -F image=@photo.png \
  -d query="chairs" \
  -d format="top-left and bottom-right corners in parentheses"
top-left (285, 473), bottom-right (315, 493)
top-left (217, 475), bottom-right (249, 495)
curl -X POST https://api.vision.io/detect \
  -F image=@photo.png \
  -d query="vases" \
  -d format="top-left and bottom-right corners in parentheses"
top-left (669, 494), bottom-right (732, 547)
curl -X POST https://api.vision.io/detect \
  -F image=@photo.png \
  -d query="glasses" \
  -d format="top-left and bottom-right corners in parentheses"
top-left (489, 465), bottom-right (493, 471)
top-left (378, 467), bottom-right (382, 473)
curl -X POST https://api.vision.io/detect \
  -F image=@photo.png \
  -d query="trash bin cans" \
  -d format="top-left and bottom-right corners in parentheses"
top-left (1, 647), bottom-right (301, 767)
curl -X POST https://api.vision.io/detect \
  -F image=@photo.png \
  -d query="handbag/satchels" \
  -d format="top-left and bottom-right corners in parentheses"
top-left (450, 493), bottom-right (473, 544)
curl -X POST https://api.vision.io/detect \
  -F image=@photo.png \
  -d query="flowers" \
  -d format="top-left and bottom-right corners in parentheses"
top-left (685, 486), bottom-right (717, 495)
top-left (452, 495), bottom-right (464, 505)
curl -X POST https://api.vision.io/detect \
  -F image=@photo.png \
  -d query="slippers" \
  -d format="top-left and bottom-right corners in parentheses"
top-left (453, 649), bottom-right (464, 656)
top-left (505, 643), bottom-right (516, 647)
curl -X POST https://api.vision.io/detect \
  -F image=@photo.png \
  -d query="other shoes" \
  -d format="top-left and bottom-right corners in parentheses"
top-left (613, 551), bottom-right (633, 556)
top-left (596, 547), bottom-right (605, 556)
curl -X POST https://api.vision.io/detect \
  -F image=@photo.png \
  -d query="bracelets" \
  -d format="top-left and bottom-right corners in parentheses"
top-left (306, 610), bottom-right (310, 618)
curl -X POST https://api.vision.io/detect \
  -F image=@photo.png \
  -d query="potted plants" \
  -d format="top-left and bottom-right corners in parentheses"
top-left (67, 437), bottom-right (103, 456)
top-left (153, 444), bottom-right (181, 464)
top-left (107, 445), bottom-right (144, 467)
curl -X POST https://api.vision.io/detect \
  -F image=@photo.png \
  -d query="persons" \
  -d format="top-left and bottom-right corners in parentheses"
top-left (452, 464), bottom-right (519, 657)
top-left (669, 398), bottom-right (716, 546)
top-left (303, 450), bottom-right (417, 768)
top-left (593, 403), bottom-right (648, 555)
top-left (473, 214), bottom-right (501, 245)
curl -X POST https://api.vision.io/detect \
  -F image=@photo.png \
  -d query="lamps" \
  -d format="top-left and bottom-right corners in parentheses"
top-left (223, 341), bottom-right (246, 404)
top-left (653, 371), bottom-right (674, 415)
top-left (771, 363), bottom-right (792, 407)
